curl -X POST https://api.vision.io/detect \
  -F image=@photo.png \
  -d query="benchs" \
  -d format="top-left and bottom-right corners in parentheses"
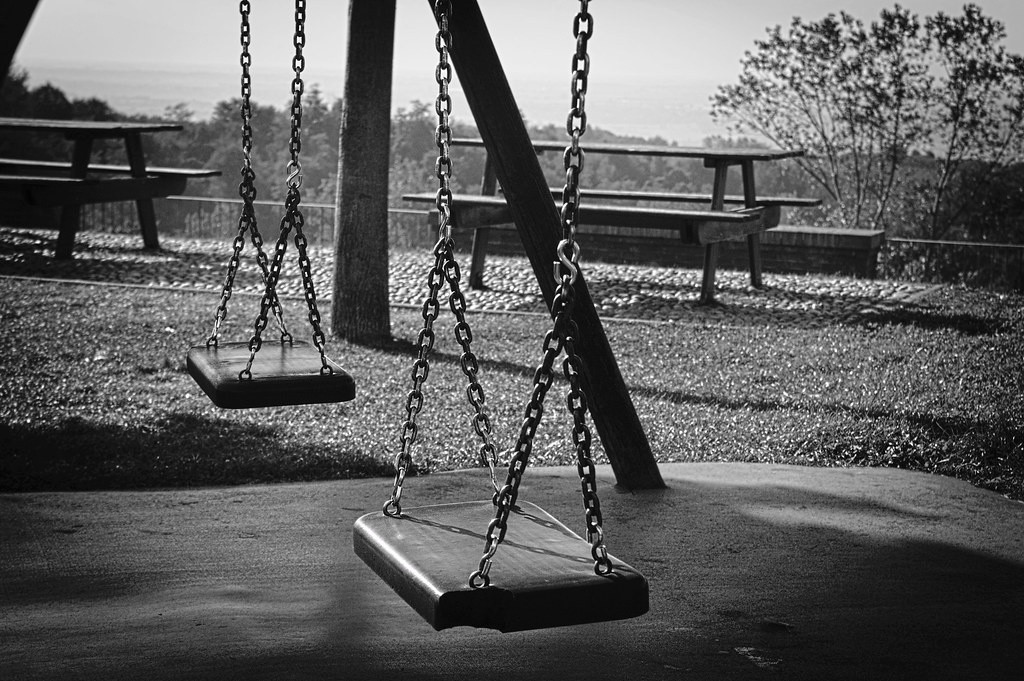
top-left (401, 192), bottom-right (761, 249)
top-left (497, 186), bottom-right (823, 229)
top-left (0, 174), bottom-right (100, 208)
top-left (0, 157), bottom-right (222, 196)
top-left (427, 206), bottom-right (886, 279)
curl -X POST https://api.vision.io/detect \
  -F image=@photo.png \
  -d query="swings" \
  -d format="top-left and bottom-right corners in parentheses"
top-left (352, 0), bottom-right (652, 634)
top-left (185, 0), bottom-right (357, 410)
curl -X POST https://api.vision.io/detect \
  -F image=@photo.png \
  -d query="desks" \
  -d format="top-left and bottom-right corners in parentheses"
top-left (0, 117), bottom-right (184, 262)
top-left (442, 137), bottom-right (805, 305)
